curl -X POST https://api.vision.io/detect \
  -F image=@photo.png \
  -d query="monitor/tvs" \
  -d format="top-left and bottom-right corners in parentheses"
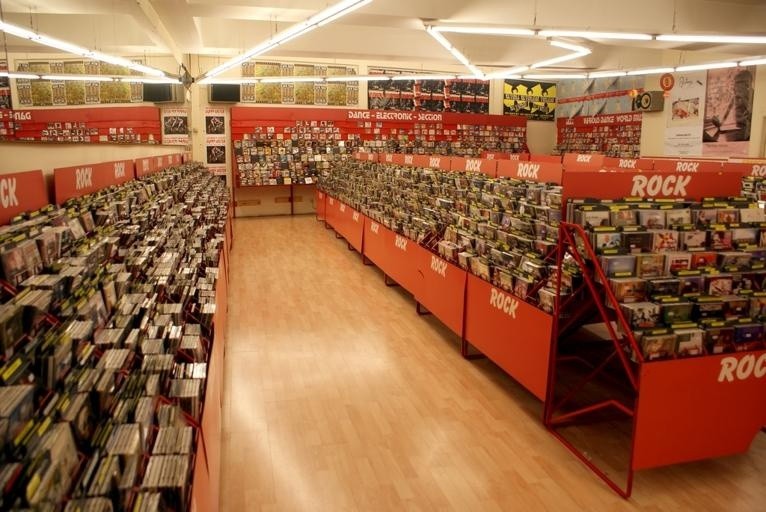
top-left (208, 82), bottom-right (241, 103)
top-left (142, 82), bottom-right (186, 104)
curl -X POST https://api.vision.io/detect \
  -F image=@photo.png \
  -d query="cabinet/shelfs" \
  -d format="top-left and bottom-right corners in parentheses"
top-left (310, 147), bottom-right (766, 503)
top-left (0, 160), bottom-right (233, 512)
